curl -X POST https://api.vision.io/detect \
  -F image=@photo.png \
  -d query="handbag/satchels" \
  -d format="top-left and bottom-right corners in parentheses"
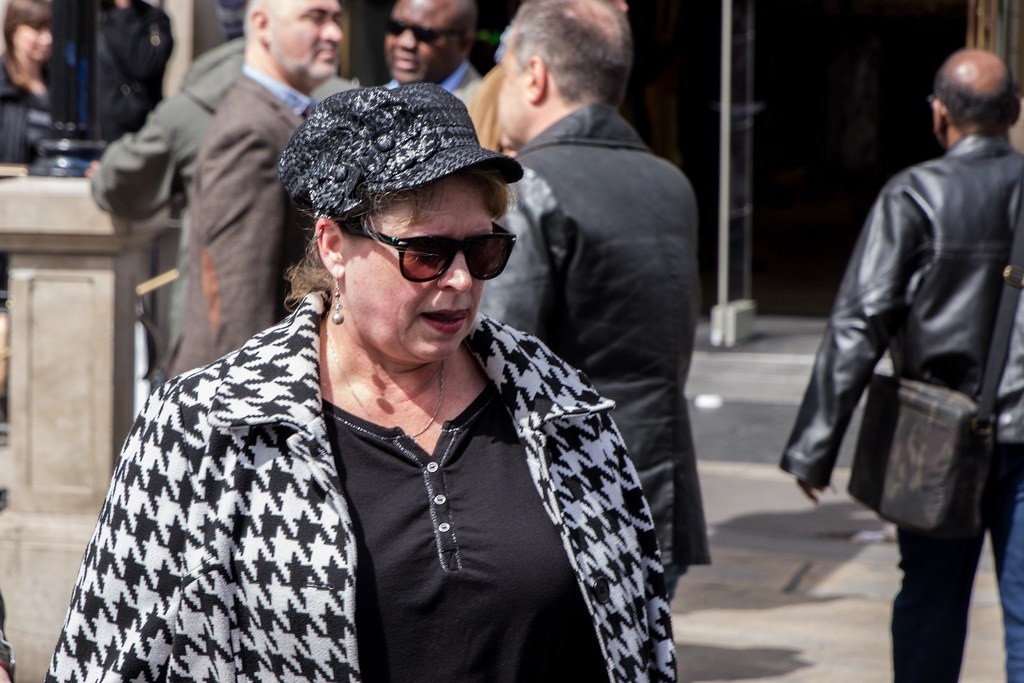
top-left (846, 372), bottom-right (999, 542)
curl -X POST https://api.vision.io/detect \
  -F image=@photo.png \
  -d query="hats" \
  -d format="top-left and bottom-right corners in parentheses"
top-left (277, 81), bottom-right (524, 218)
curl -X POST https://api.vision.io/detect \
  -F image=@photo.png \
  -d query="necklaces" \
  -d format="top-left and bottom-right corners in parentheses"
top-left (323, 311), bottom-right (446, 438)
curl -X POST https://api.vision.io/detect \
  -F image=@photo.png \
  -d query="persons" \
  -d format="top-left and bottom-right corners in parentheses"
top-left (779, 49), bottom-right (1024, 683)
top-left (47, 82), bottom-right (678, 683)
top-left (0, 1), bottom-right (714, 683)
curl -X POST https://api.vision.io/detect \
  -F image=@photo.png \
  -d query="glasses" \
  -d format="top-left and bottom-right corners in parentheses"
top-left (342, 222), bottom-right (520, 284)
top-left (381, 16), bottom-right (457, 45)
